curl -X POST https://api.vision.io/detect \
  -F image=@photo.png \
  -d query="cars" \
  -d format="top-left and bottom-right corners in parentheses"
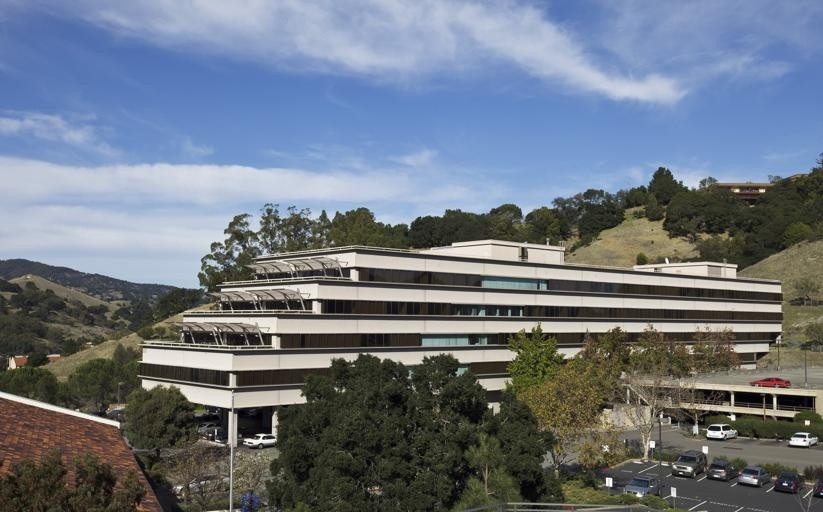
top-left (750, 378), bottom-right (791, 388)
top-left (196, 422), bottom-right (227, 442)
top-left (706, 460), bottom-right (823, 498)
top-left (789, 432), bottom-right (819, 449)
top-left (171, 474), bottom-right (233, 498)
top-left (243, 433), bottom-right (276, 449)
top-left (107, 410), bottom-right (129, 423)
top-left (706, 423), bottom-right (738, 441)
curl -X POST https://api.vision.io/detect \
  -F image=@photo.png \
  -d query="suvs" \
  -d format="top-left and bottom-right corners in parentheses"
top-left (670, 449), bottom-right (707, 479)
top-left (622, 476), bottom-right (663, 500)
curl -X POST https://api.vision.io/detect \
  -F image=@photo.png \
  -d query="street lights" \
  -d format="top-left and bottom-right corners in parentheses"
top-left (117, 382), bottom-right (126, 407)
